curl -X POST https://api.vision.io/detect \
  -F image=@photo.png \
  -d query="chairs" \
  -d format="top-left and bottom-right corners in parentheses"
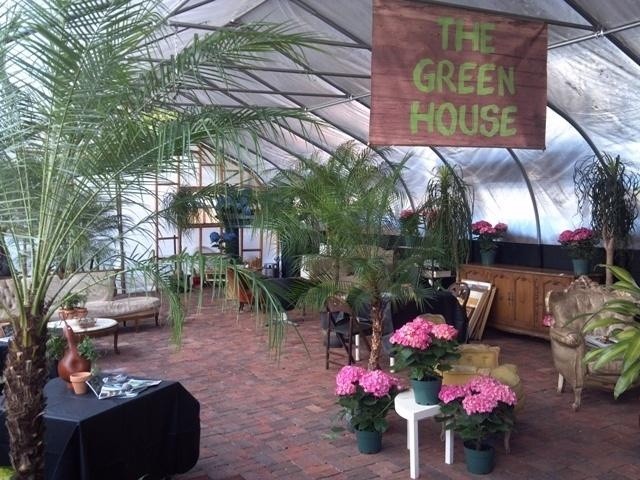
top-left (323, 295), bottom-right (371, 370)
top-left (545, 275), bottom-right (636, 411)
top-left (447, 281), bottom-right (470, 308)
top-left (434, 343), bottom-right (525, 456)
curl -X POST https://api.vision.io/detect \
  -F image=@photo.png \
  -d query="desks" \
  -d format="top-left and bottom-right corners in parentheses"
top-left (0, 371), bottom-right (200, 480)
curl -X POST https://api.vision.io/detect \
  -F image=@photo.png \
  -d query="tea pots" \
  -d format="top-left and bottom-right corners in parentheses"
top-left (87, 316), bottom-right (97, 327)
top-left (77, 316), bottom-right (88, 329)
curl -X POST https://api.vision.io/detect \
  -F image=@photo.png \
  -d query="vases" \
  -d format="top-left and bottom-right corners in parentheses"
top-left (481, 252), bottom-right (496, 265)
top-left (404, 234), bottom-right (416, 257)
top-left (353, 423), bottom-right (382, 454)
top-left (463, 440), bottom-right (495, 474)
top-left (409, 376), bottom-right (444, 406)
top-left (70, 371), bottom-right (92, 395)
top-left (572, 258), bottom-right (593, 277)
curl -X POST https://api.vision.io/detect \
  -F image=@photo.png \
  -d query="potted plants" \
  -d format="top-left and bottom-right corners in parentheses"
top-left (264, 139), bottom-right (393, 348)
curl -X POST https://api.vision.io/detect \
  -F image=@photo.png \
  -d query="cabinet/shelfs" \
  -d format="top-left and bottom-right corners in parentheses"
top-left (460, 263), bottom-right (574, 341)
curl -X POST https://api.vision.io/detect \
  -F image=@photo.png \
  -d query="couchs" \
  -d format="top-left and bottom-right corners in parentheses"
top-left (0, 269), bottom-right (162, 333)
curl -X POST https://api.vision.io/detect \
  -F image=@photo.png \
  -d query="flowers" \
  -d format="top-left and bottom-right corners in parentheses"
top-left (388, 316), bottom-right (459, 381)
top-left (434, 374), bottom-right (518, 449)
top-left (471, 220), bottom-right (508, 252)
top-left (331, 365), bottom-right (407, 432)
top-left (557, 226), bottom-right (599, 258)
top-left (399, 210), bottom-right (420, 233)
